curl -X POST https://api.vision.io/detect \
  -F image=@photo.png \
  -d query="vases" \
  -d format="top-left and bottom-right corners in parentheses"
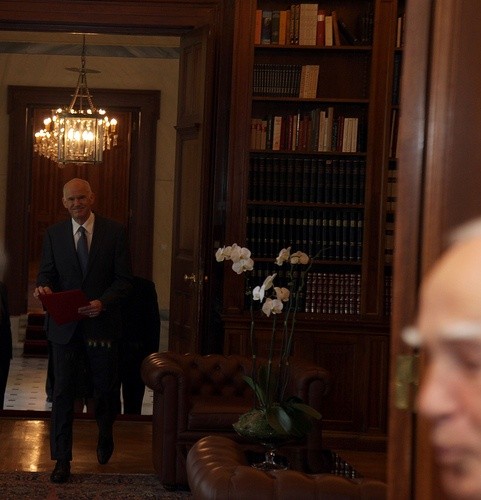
top-left (249, 432), bottom-right (289, 471)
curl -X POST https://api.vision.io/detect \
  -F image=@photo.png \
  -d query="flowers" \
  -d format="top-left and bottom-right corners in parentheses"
top-left (215, 243), bottom-right (331, 438)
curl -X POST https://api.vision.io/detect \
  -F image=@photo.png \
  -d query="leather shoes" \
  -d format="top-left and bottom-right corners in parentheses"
top-left (97, 426), bottom-right (114, 464)
top-left (51, 459), bottom-right (70, 483)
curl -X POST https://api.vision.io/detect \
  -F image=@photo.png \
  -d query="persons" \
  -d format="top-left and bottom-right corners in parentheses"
top-left (0, 285), bottom-right (13, 419)
top-left (401, 217), bottom-right (481, 500)
top-left (32, 178), bottom-right (130, 485)
top-left (115, 273), bottom-right (162, 419)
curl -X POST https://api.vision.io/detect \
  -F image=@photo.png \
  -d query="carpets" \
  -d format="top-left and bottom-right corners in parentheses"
top-left (0, 471), bottom-right (194, 500)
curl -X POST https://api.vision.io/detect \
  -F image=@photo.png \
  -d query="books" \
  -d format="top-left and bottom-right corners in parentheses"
top-left (250, 106), bottom-right (367, 153)
top-left (255, 2), bottom-right (373, 45)
top-left (382, 13), bottom-right (405, 319)
top-left (38, 287), bottom-right (92, 327)
top-left (240, 270), bottom-right (361, 317)
top-left (252, 61), bottom-right (320, 99)
top-left (244, 206), bottom-right (364, 262)
top-left (246, 157), bottom-right (366, 201)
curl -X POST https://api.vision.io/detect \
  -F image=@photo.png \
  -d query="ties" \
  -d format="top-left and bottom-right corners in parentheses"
top-left (77, 226), bottom-right (89, 282)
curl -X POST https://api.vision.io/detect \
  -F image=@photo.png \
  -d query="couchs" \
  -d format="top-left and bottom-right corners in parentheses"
top-left (185, 437), bottom-right (386, 500)
top-left (140, 352), bottom-right (332, 491)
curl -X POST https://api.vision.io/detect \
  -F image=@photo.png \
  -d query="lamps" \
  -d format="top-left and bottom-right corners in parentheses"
top-left (57, 32), bottom-right (104, 164)
top-left (34, 67), bottom-right (118, 167)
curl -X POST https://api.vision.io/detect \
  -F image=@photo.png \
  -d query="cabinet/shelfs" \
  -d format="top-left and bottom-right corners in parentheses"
top-left (201, 0), bottom-right (406, 452)
